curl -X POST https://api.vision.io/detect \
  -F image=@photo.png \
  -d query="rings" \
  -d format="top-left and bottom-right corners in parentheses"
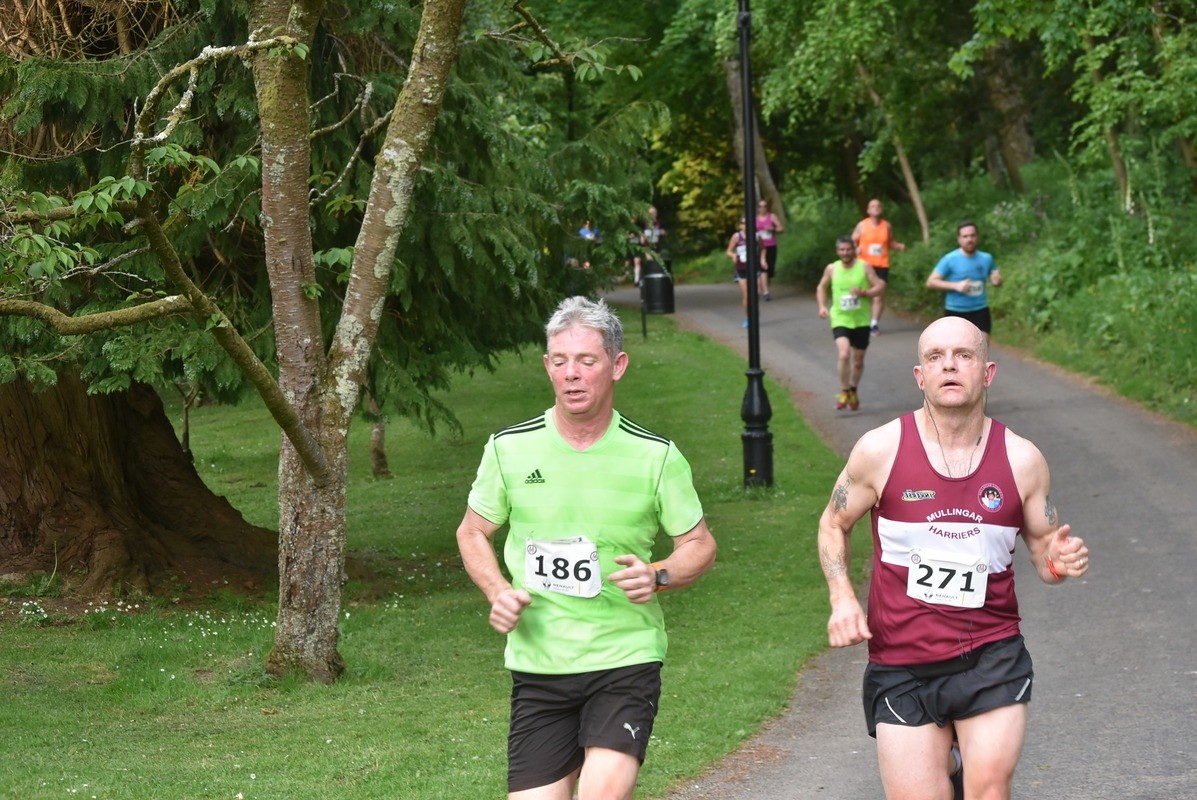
top-left (1079, 559), bottom-right (1084, 567)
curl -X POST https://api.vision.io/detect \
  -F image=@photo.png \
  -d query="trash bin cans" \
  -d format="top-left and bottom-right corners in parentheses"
top-left (642, 259), bottom-right (673, 313)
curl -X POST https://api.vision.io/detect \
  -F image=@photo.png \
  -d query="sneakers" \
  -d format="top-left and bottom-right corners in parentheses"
top-left (742, 320), bottom-right (749, 329)
top-left (764, 291), bottom-right (771, 301)
top-left (848, 391), bottom-right (858, 411)
top-left (870, 324), bottom-right (879, 337)
top-left (836, 389), bottom-right (849, 410)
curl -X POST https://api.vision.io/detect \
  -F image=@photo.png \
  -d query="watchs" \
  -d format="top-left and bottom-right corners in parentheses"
top-left (652, 562), bottom-right (670, 593)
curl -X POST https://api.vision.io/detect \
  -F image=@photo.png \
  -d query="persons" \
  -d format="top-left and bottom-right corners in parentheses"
top-left (818, 316), bottom-right (1089, 800)
top-left (455, 295), bottom-right (719, 800)
top-left (849, 199), bottom-right (906, 336)
top-left (924, 221), bottom-right (1001, 346)
top-left (752, 198), bottom-right (782, 302)
top-left (726, 216), bottom-right (769, 330)
top-left (816, 235), bottom-right (887, 410)
top-left (576, 205), bottom-right (668, 289)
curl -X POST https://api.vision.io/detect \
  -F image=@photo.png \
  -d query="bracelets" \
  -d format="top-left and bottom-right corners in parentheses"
top-left (1045, 551), bottom-right (1064, 584)
